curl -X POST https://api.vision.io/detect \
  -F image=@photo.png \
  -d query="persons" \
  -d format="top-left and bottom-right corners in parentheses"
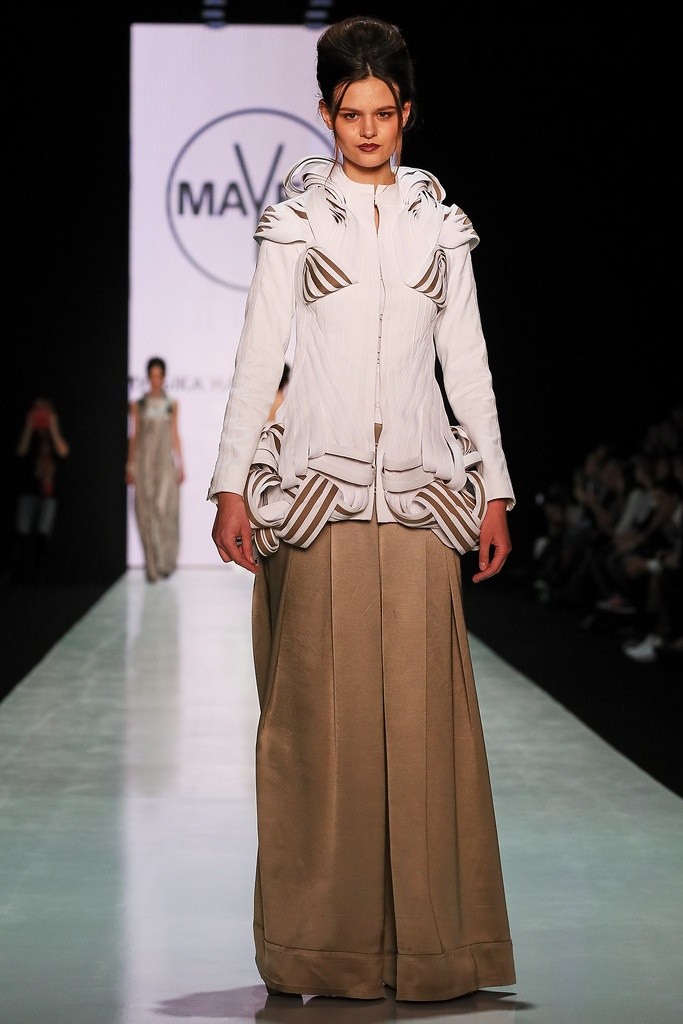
top-left (536, 407), bottom-right (683, 654)
top-left (268, 363), bottom-right (290, 423)
top-left (128, 358), bottom-right (186, 583)
top-left (21, 400), bottom-right (71, 533)
top-left (207, 15), bottom-right (517, 1003)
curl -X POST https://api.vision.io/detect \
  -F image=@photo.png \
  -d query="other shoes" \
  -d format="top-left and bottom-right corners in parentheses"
top-left (623, 632), bottom-right (661, 661)
top-left (596, 593), bottom-right (638, 616)
